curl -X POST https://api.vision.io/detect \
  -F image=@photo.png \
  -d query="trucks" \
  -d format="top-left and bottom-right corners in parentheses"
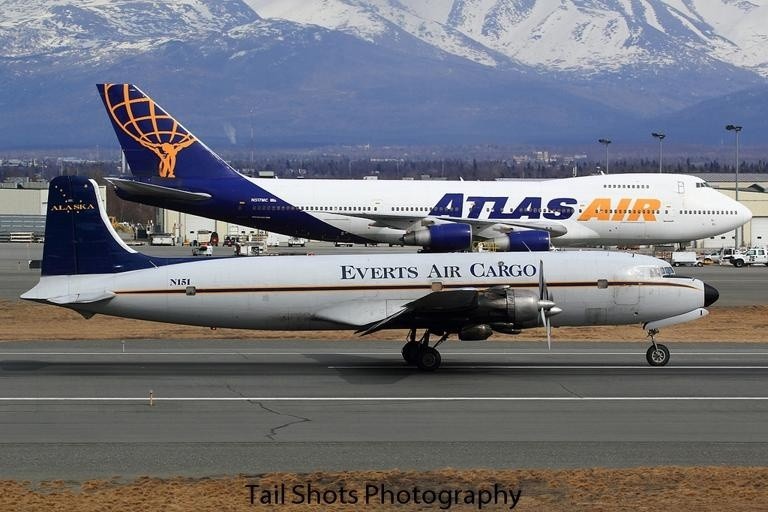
top-left (669, 251), bottom-right (705, 268)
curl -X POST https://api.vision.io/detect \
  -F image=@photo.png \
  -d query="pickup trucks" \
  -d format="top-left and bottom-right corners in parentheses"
top-left (730, 248), bottom-right (768, 269)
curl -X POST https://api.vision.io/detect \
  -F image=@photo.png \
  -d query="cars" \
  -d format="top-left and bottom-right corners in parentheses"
top-left (703, 249), bottom-right (723, 263)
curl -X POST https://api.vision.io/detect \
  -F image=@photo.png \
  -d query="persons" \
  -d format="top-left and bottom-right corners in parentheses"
top-left (234, 238), bottom-right (241, 255)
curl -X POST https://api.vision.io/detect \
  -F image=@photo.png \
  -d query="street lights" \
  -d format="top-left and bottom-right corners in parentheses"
top-left (597, 139), bottom-right (612, 175)
top-left (650, 131), bottom-right (667, 174)
top-left (724, 123), bottom-right (745, 253)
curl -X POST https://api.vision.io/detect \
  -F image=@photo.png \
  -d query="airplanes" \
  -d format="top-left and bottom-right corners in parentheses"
top-left (91, 81), bottom-right (755, 286)
top-left (15, 173), bottom-right (721, 375)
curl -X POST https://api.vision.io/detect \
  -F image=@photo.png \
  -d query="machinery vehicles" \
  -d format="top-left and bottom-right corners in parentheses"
top-left (107, 215), bottom-right (306, 260)
